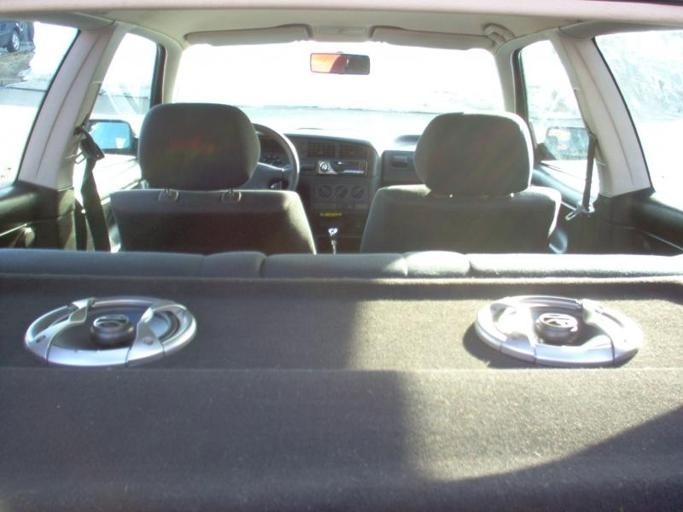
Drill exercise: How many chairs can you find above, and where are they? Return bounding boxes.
[359,111,563,255]
[108,100,313,257]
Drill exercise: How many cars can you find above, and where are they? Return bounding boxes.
[0,16,35,53]
